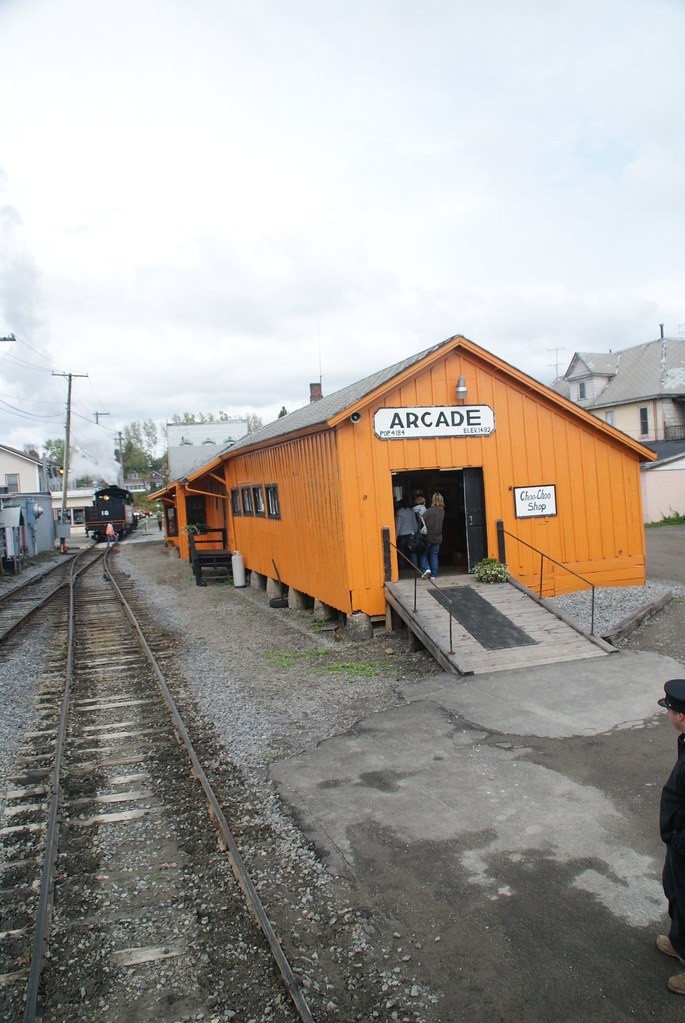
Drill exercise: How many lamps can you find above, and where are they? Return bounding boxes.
[455,377,467,400]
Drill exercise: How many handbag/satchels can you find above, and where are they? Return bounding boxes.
[408,519,428,554]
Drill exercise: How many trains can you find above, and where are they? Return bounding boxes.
[84,485,134,541]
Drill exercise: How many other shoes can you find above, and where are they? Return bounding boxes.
[667,974,685,994]
[431,574,435,579]
[656,934,685,965]
[421,568,431,579]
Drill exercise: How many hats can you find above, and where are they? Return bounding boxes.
[657,679,685,714]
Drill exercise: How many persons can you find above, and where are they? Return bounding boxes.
[394,499,417,571]
[420,493,445,580]
[656,679,685,995]
[412,497,427,515]
[106,521,113,547]
[157,511,162,531]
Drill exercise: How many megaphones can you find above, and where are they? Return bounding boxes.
[350,411,361,423]
